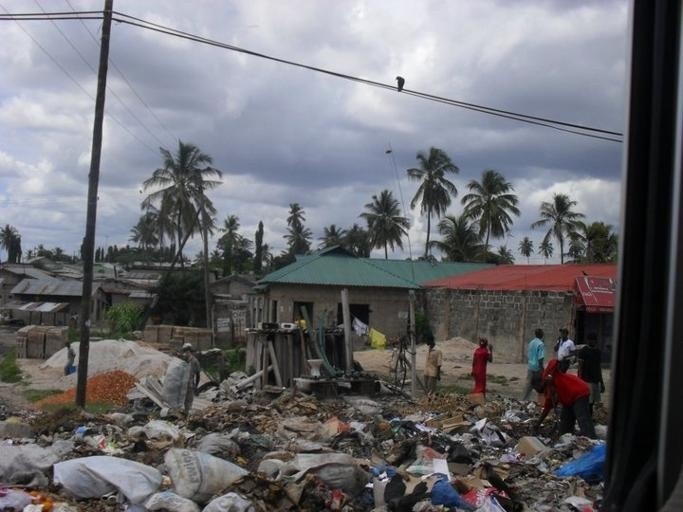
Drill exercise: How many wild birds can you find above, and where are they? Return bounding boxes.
[581,270,592,276]
[384,472,405,502]
[455,402,506,451]
[394,75,405,93]
[479,460,514,498]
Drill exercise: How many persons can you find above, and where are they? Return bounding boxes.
[518,326,545,407]
[528,368,597,443]
[551,327,573,373]
[422,338,443,394]
[537,357,570,407]
[468,335,493,399]
[60,341,76,376]
[181,341,202,420]
[574,333,605,419]
